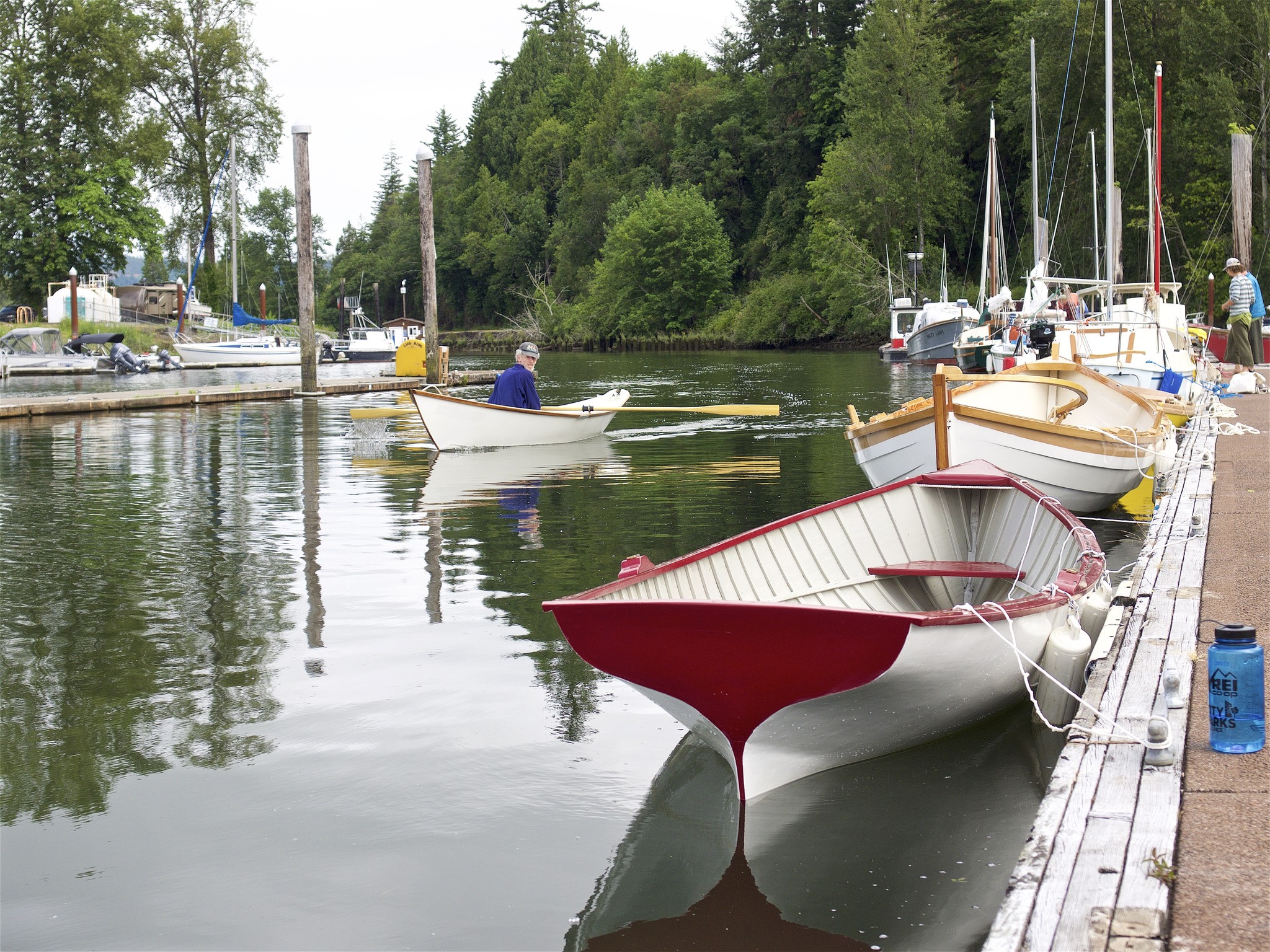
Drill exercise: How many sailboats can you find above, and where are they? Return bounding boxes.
[164,137,340,369]
[876,0,1221,419]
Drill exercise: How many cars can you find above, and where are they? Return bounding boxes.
[0,304,38,322]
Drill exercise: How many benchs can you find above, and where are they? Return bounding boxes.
[868,561,1026,580]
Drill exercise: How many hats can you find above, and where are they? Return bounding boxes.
[1060,284,1073,292]
[519,342,539,359]
[1222,257,1241,271]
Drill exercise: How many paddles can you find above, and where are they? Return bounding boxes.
[349,408,418,420]
[540,405,779,416]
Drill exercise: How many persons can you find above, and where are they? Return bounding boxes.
[1009,314,1027,346]
[1221,257,1267,375]
[487,342,541,411]
[1054,285,1089,322]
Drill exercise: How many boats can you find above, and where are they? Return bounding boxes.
[0,327,99,376]
[314,270,410,363]
[524,459,1113,804]
[133,345,185,372]
[845,340,1180,526]
[409,387,632,454]
[62,332,150,376]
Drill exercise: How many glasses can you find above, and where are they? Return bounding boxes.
[1225,268,1229,273]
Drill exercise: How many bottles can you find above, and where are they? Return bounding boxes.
[1194,618,1266,754]
